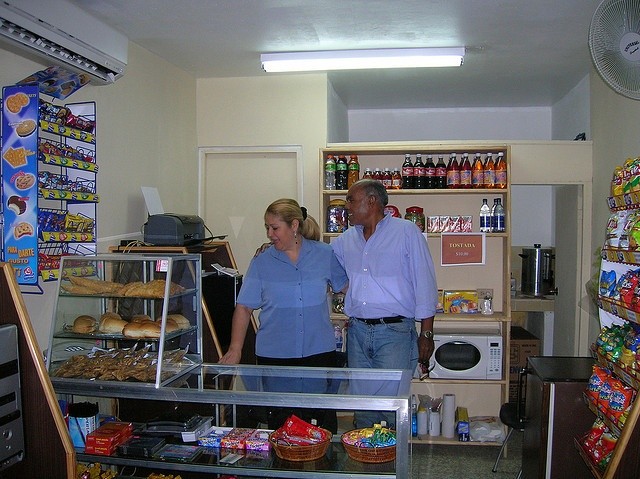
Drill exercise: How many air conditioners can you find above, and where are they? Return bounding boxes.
[1,1,129,86]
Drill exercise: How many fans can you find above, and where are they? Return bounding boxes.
[588,0,640,100]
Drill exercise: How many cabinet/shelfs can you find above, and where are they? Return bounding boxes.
[44,253,202,389]
[318,145,512,460]
[109,240,259,366]
[570,156,640,475]
[1,84,98,293]
[52,362,413,479]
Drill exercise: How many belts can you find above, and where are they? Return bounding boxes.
[356,315,408,324]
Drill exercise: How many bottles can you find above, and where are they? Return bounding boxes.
[424,155,436,188]
[402,154,414,188]
[447,152,459,188]
[324,155,336,189]
[335,154,348,189]
[460,152,472,188]
[363,167,401,189]
[484,152,496,188]
[435,154,447,189]
[479,198,491,233]
[496,152,507,188]
[414,154,425,188]
[347,155,359,189]
[473,152,484,188]
[491,198,505,232]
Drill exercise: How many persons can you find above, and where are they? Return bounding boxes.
[215,199,348,471]
[253,179,438,432]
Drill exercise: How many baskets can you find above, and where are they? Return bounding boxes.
[268,426,332,462]
[340,427,396,464]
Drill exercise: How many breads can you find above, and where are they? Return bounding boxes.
[6,195,26,217]
[70,309,191,339]
[61,273,186,298]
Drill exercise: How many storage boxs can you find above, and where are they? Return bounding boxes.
[517,356,639,478]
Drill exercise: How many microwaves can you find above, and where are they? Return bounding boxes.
[428,327,503,380]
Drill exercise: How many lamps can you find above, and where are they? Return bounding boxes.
[259,45,465,73]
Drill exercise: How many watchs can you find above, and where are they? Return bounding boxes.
[421,329,434,338]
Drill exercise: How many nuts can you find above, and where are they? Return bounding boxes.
[55,344,192,381]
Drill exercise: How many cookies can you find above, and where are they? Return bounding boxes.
[15,92,30,107]
[15,119,36,137]
[6,96,23,113]
[15,222,34,240]
[17,171,36,191]
[4,145,28,168]
